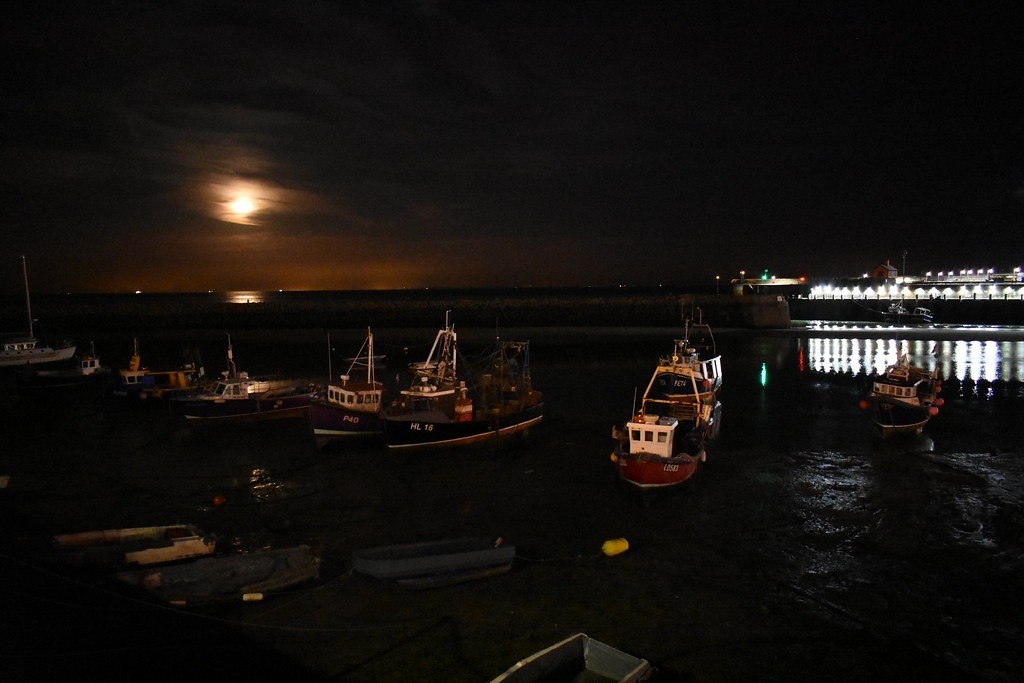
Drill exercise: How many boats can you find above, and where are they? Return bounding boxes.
[0,254,77,370]
[271,325,394,437]
[867,300,938,328]
[136,547,320,606]
[735,270,807,330]
[611,307,726,498]
[78,336,325,431]
[862,354,955,441]
[486,632,660,683]
[345,532,515,592]
[53,524,219,563]
[382,308,549,451]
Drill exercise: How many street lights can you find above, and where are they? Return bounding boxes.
[715,274,721,294]
